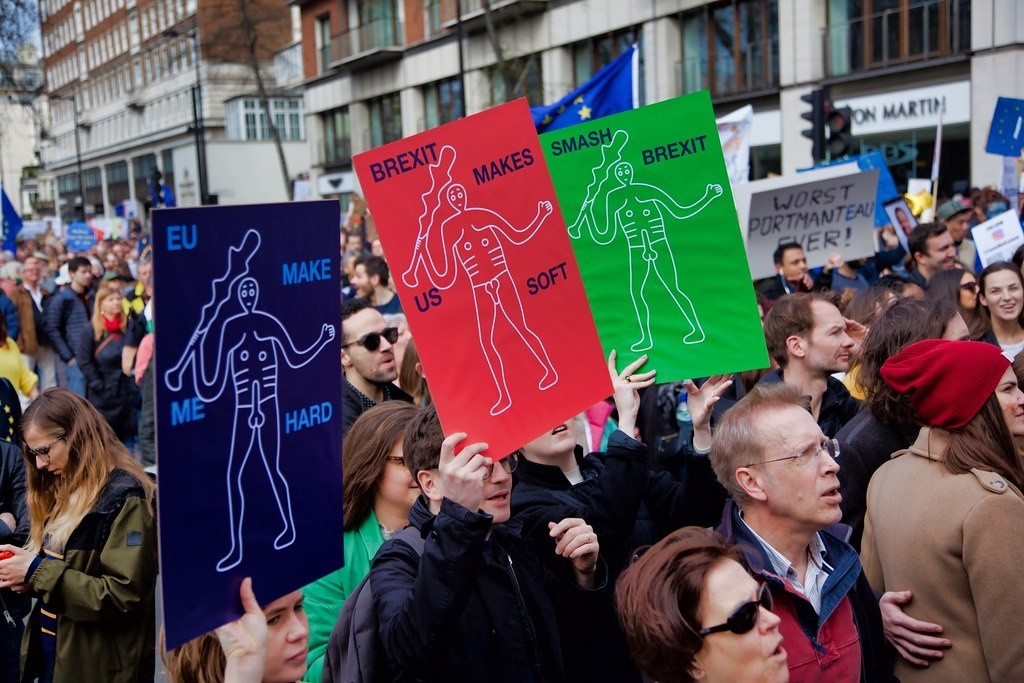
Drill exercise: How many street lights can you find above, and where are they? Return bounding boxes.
[161,28,209,207]
[48,92,85,223]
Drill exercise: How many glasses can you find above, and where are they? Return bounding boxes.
[698,582,773,636]
[733,438,840,475]
[29,432,66,455]
[340,327,399,352]
[416,449,519,486]
[959,282,977,293]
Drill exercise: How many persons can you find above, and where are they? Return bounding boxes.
[0,188,1024,683]
[894,207,913,236]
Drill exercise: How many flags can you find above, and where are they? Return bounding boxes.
[530,44,639,135]
[0,186,23,254]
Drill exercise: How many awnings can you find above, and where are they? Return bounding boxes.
[329,45,403,73]
[443,0,548,36]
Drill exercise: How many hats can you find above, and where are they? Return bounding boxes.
[880,338,1011,429]
[937,202,973,221]
[55,264,72,285]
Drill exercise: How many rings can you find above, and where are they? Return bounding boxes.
[586,534,590,543]
[626,376,631,382]
[712,392,714,397]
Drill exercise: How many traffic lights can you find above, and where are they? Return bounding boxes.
[801,88,831,163]
[828,106,853,163]
